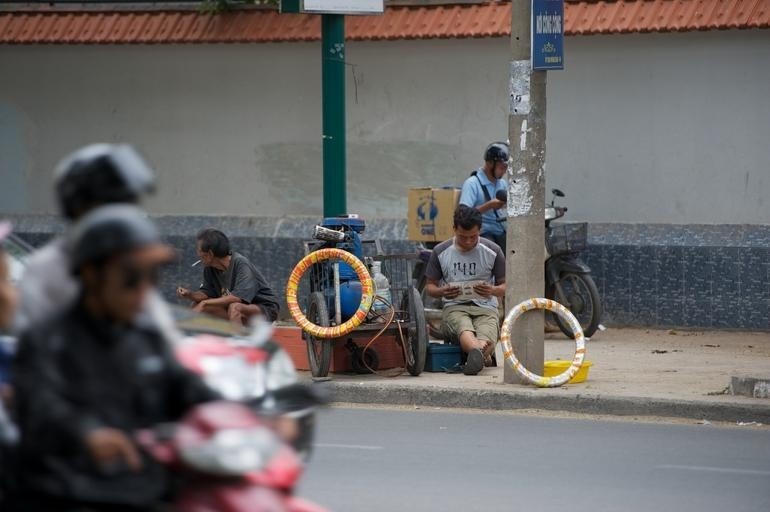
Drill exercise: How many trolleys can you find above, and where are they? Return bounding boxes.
[299,219,431,381]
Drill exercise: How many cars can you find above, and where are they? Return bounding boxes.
[2,232,327,467]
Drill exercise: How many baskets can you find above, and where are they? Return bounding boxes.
[544,222,588,257]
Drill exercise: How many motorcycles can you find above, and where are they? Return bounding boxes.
[409,188,603,338]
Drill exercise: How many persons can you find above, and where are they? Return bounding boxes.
[458,143,562,332]
[173,226,278,327]
[0,219,16,338]
[12,143,166,337]
[17,203,290,511]
[426,207,507,374]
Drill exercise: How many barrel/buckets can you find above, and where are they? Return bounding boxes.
[369,265,392,311]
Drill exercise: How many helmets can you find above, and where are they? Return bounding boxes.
[48,140,161,218]
[483,140,510,164]
[65,201,165,275]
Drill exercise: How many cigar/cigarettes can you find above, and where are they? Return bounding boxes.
[191,260,201,267]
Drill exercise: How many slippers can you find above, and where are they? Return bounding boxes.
[462,347,484,376]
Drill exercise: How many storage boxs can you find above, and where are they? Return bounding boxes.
[407,183,462,241]
